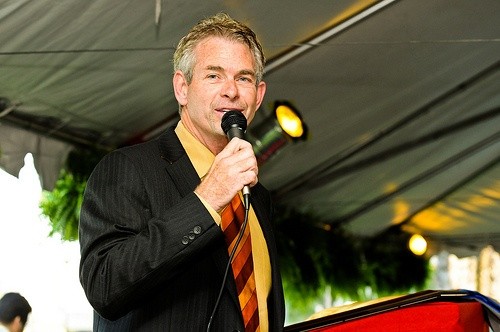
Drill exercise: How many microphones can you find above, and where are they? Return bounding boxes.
[220,111,249,197]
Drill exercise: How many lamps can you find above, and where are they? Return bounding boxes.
[242,99,308,167]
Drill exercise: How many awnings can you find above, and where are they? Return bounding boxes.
[0,0,500,265]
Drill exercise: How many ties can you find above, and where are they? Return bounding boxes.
[217,193,262,332]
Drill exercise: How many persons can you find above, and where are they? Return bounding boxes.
[0,293,33,332]
[78,12,287,331]
